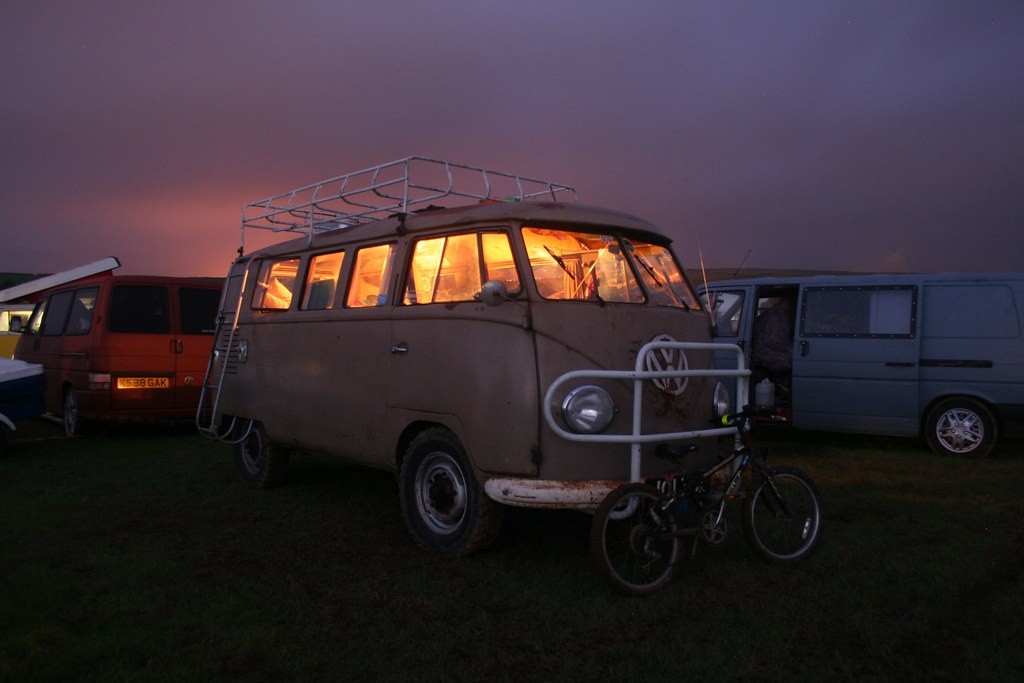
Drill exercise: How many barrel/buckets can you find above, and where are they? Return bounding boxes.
[755,379,775,406]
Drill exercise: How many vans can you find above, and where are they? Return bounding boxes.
[690,273,1024,461]
[195,158,729,554]
[10,272,230,436]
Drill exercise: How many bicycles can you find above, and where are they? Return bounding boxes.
[588,406,826,594]
